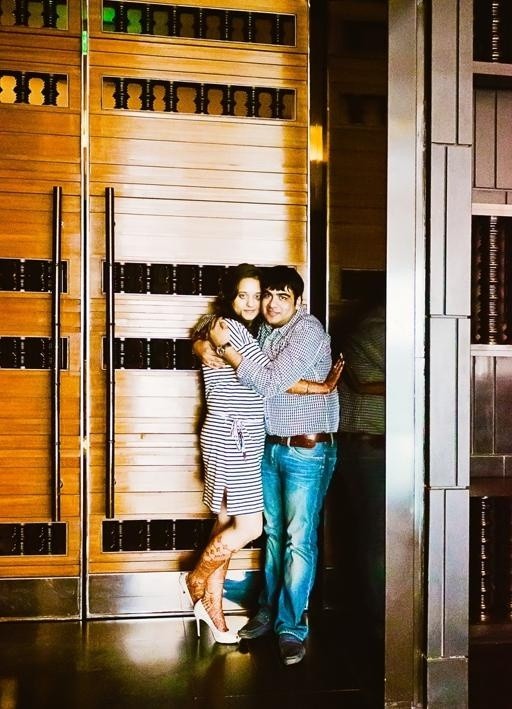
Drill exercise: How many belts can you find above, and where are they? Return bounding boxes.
[265,433,331,448]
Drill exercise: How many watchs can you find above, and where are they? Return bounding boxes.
[216,342,232,357]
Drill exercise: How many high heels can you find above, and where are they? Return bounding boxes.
[179,571,241,643]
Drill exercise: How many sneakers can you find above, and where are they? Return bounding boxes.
[238,608,275,640]
[278,634,306,666]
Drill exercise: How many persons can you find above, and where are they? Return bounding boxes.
[177,264,347,646]
[190,264,341,667]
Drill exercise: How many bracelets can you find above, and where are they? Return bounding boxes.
[307,381,310,394]
